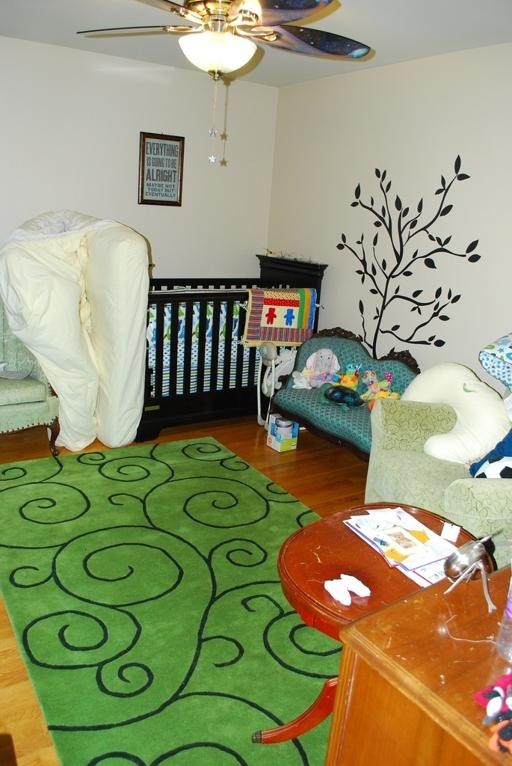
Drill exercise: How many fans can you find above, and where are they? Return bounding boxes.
[76,1,371,61]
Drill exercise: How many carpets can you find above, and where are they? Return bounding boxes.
[1,435,343,766]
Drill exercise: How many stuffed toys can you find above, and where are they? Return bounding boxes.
[358,370,393,403]
[261,347,298,398]
[368,388,402,412]
[300,348,341,389]
[330,364,361,392]
[291,370,313,390]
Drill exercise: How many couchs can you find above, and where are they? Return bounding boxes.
[272,326,422,463]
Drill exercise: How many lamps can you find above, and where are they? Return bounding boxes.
[256,342,282,432]
[177,31,259,164]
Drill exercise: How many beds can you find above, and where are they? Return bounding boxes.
[136,255,329,444]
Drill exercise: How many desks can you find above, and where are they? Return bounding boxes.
[250,502,494,743]
[324,563,512,766]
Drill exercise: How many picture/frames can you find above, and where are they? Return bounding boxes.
[137,131,184,207]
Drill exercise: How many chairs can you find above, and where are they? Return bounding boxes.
[364,397,512,570]
[0,302,60,457]
